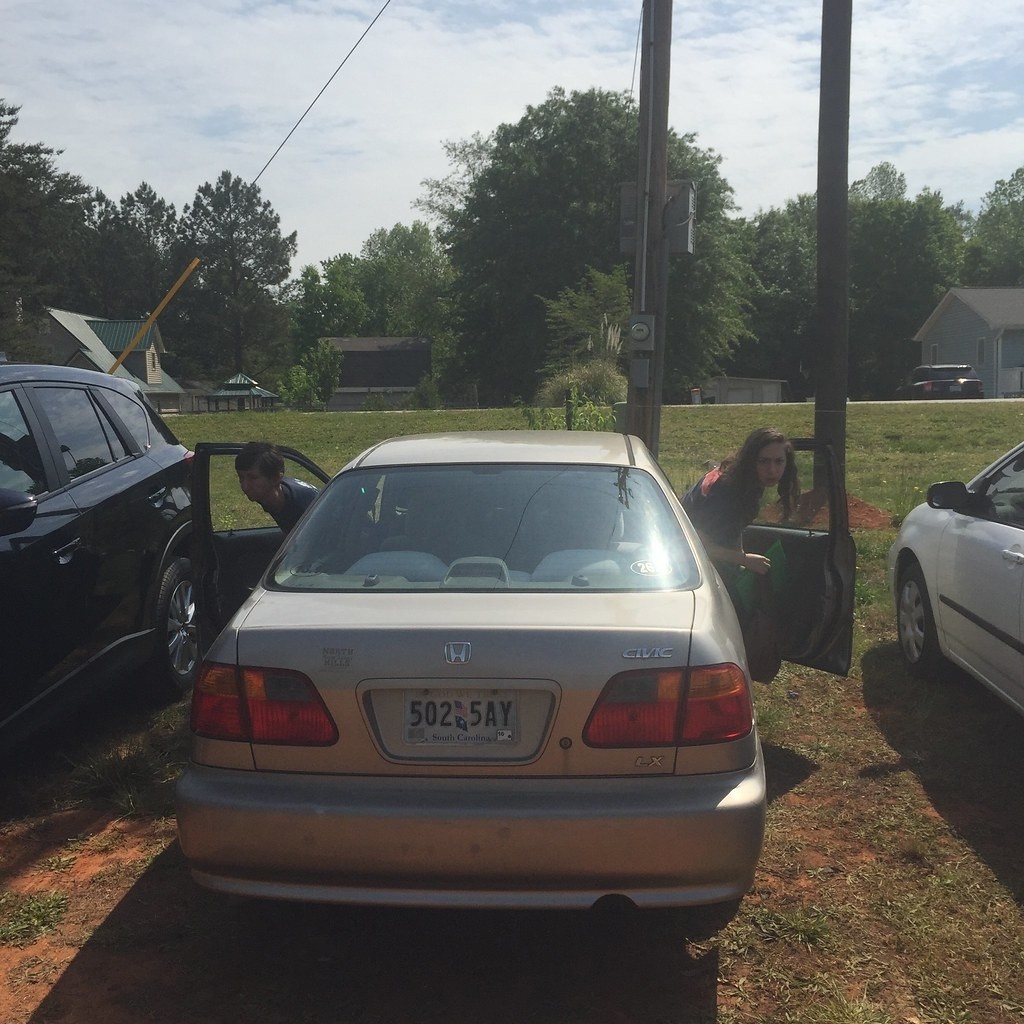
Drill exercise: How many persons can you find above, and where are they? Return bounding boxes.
[233,442,321,537]
[677,426,802,611]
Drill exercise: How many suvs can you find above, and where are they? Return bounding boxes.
[894,362,985,399]
[0,364,204,763]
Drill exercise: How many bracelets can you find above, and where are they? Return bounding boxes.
[741,553,747,566]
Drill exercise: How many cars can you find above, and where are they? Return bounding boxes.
[173,429,856,915]
[887,437,1024,716]
